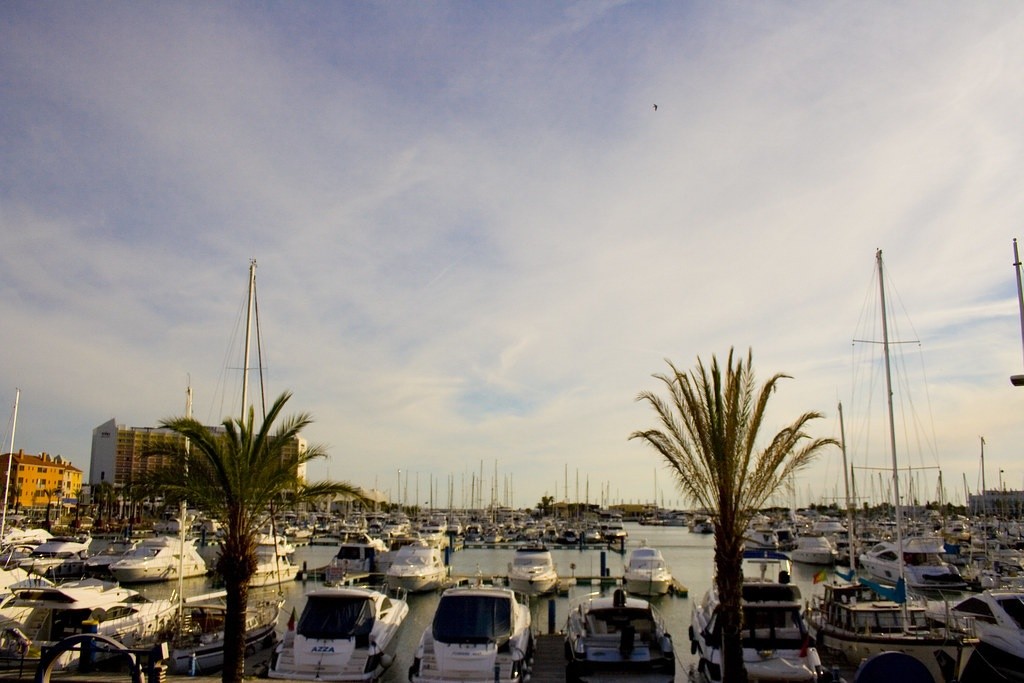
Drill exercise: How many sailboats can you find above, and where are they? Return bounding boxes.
[802,250,981,682]
[687,403,1024,592]
[152,387,285,676]
[283,459,628,552]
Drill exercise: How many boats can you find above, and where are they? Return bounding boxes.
[82,537,141,574]
[386,538,452,592]
[622,538,672,596]
[247,525,301,586]
[19,536,92,578]
[337,524,390,570]
[408,566,537,683]
[108,536,209,582]
[0,576,177,670]
[267,584,409,682]
[561,588,675,683]
[689,578,833,683]
[912,591,1024,671]
[506,538,558,595]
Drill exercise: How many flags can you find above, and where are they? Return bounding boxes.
[813,569,828,584]
